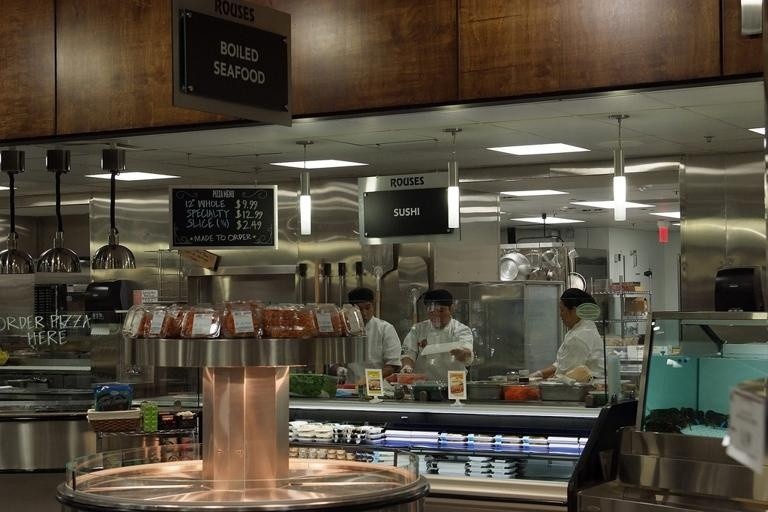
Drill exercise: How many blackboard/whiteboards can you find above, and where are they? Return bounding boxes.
[168,184,278,250]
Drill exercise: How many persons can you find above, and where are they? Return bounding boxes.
[327,287,402,379]
[525,288,605,381]
[400,289,475,379]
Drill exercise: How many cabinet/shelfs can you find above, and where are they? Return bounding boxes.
[586,275,654,360]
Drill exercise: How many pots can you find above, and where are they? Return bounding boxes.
[497,246,588,294]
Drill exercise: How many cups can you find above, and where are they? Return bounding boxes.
[519,369,530,383]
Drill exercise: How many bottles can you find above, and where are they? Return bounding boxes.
[506,368,517,380]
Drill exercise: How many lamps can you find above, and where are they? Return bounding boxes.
[606,114,637,221]
[295,139,314,236]
[444,127,463,228]
[0,148,142,273]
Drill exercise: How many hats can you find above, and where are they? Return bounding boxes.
[348,287,374,304]
[561,288,597,307]
[423,289,452,306]
